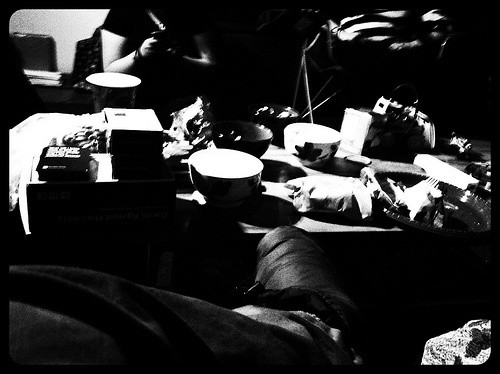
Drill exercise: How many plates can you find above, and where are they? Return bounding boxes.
[366,171,492,238]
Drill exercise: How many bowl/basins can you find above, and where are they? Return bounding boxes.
[247,103,299,142]
[283,122,342,170]
[212,121,273,160]
[187,147,265,212]
[241,161,308,215]
[86,72,143,112]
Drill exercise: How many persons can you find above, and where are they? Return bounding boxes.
[8,225,370,366]
[99,9,218,106]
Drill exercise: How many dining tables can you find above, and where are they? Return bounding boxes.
[9,113,493,240]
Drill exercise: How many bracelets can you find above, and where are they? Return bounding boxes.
[133,46,146,66]
